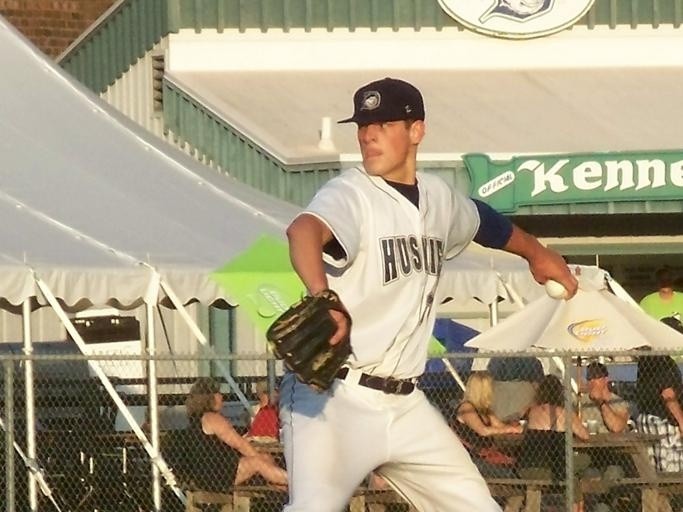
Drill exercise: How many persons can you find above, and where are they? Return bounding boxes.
[267,78,578,512]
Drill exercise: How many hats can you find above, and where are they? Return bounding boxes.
[586,362,608,379]
[337,77,425,123]
[650,264,679,282]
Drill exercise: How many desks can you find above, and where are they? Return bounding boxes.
[489,431,671,478]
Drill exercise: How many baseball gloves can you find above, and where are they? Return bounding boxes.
[266,289,357,393]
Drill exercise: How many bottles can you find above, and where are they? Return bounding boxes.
[587,414,598,436]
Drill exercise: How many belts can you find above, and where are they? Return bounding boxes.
[335,367,414,394]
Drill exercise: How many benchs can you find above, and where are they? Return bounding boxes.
[181,473,681,511]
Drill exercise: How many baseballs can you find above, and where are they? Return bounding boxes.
[545,279,568,299]
